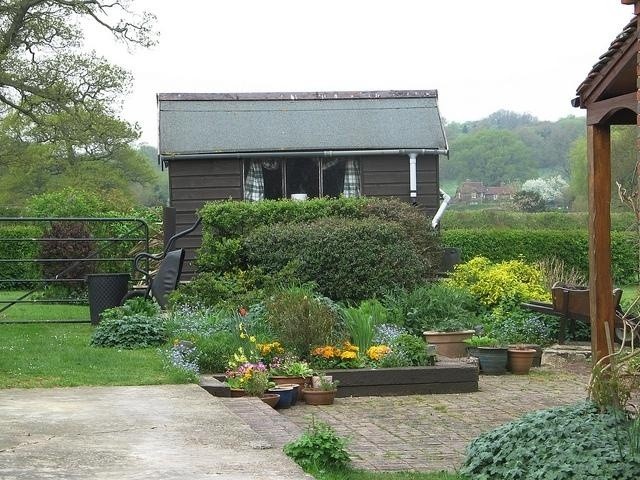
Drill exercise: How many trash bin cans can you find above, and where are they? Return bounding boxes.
[88,273,128,327]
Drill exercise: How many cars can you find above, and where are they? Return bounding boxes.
[543,199,569,213]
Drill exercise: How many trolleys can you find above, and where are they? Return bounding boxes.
[521,281,640,350]
[119,215,202,311]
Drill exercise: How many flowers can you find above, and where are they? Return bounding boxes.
[270,352,314,376]
[225,349,268,394]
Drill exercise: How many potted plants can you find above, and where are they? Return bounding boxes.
[420,295,543,375]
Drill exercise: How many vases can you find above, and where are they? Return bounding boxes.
[230,376,340,410]
